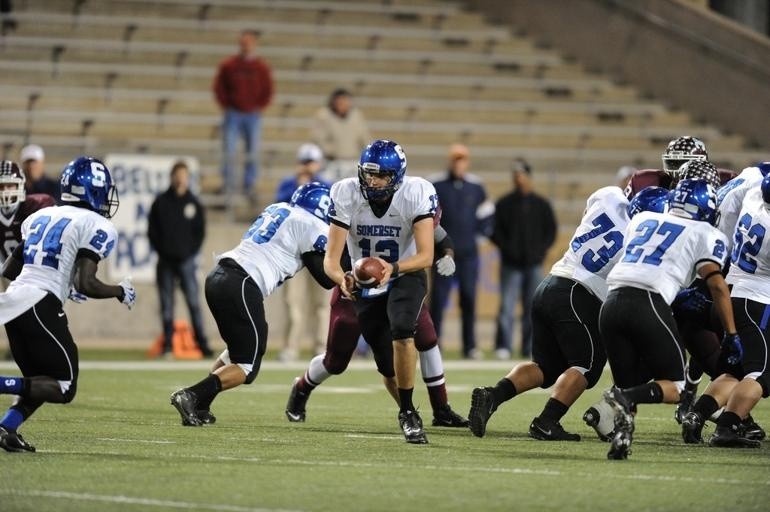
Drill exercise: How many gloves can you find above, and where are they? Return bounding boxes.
[435,255,456,277]
[68,285,89,304]
[720,331,745,365]
[675,286,709,315]
[117,275,136,310]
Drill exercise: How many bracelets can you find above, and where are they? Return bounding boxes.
[390,263,399,278]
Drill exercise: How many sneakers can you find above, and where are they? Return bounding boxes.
[675,386,765,448]
[397,387,493,444]
[0,424,35,452]
[286,376,309,421]
[529,417,581,442]
[169,387,203,426]
[196,407,216,423]
[583,383,635,459]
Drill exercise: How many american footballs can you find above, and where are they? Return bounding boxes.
[352,257,385,288]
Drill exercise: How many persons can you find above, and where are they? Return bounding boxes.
[413,211,470,427]
[467,134,770,463]
[209,26,275,207]
[0,158,57,284]
[15,144,66,207]
[169,181,350,425]
[308,86,370,182]
[430,147,493,359]
[276,143,334,359]
[0,155,135,455]
[146,158,219,364]
[287,138,439,444]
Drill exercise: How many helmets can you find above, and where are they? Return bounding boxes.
[60,155,119,218]
[290,181,337,225]
[357,140,407,203]
[0,160,28,217]
[627,136,770,220]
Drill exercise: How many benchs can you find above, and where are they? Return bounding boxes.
[0,0,770,232]
